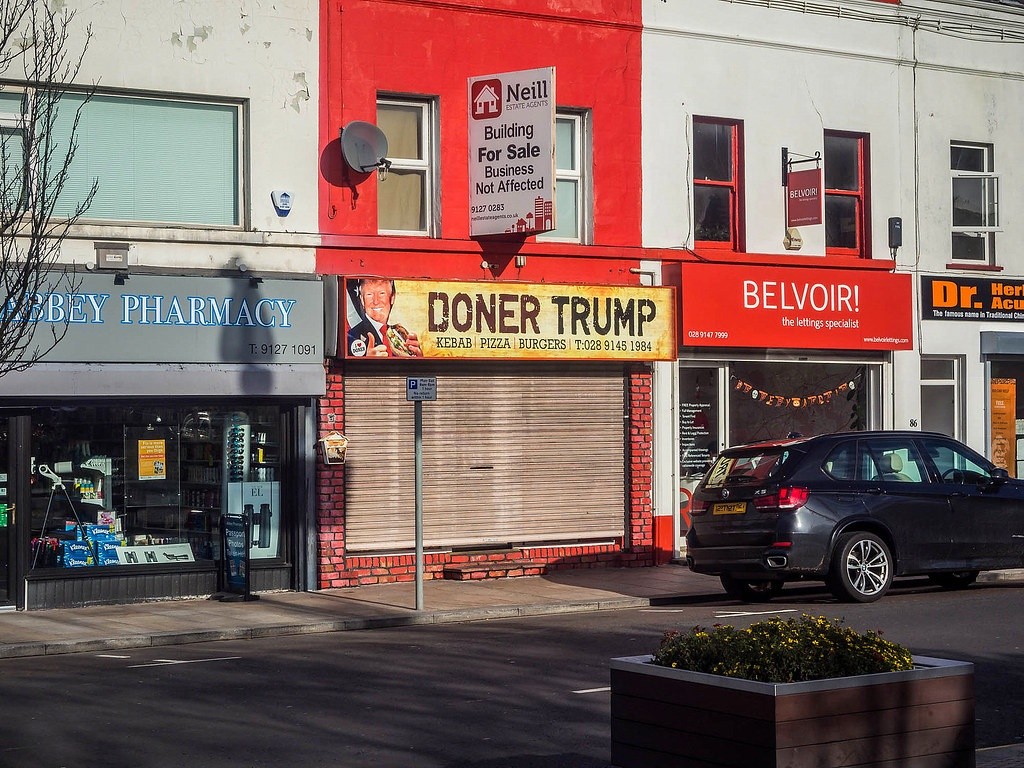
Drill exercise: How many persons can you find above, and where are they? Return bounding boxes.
[348,279,422,358]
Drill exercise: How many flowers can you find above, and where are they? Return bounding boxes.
[650,613,915,683]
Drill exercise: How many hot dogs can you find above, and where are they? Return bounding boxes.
[384,325,415,357]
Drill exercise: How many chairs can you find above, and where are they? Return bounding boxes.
[873,453,916,482]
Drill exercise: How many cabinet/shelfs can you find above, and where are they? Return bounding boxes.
[0,408,286,568]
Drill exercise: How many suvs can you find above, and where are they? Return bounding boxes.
[685,430,1024,602]
